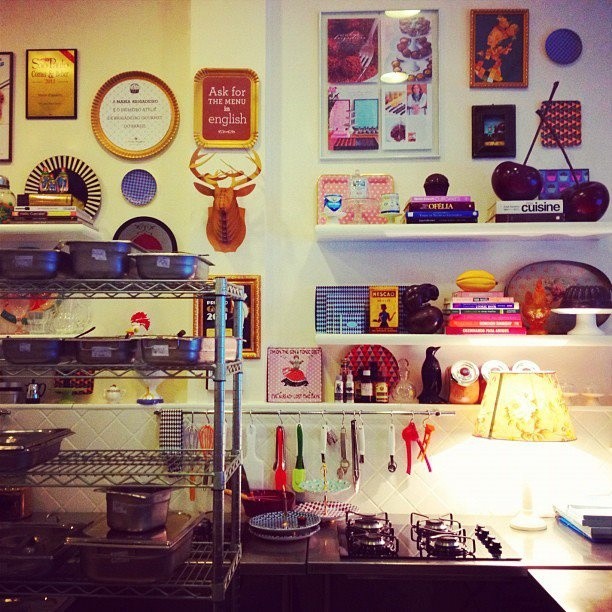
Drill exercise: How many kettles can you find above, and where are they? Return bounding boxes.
[24,379,46,404]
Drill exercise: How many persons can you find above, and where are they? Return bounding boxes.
[373,304,396,327]
[280,354,309,389]
[407,84,427,115]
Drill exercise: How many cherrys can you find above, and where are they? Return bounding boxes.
[492,81,559,200]
[534,109,609,221]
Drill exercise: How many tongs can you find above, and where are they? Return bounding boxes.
[272,424,287,490]
[351,420,360,494]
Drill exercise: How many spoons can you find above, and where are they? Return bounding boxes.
[388,423,397,473]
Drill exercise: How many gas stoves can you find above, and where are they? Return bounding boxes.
[336,510,522,561]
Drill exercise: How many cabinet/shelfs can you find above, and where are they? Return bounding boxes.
[315,221,611,349]
[0,272,246,612]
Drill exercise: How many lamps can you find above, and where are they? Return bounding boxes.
[472,370,578,533]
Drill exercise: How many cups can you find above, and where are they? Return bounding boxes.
[51,294,91,334]
[27,311,47,334]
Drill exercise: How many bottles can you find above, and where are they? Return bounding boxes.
[353,369,363,403]
[346,370,355,403]
[390,359,416,403]
[375,371,389,403]
[334,368,343,403]
[361,370,373,403]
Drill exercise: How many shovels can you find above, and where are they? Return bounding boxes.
[275,428,286,491]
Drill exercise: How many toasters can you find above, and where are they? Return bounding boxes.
[0,482,33,522]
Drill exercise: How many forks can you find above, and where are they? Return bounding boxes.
[355,18,378,82]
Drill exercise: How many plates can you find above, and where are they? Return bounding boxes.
[113,216,177,253]
[24,155,101,219]
[342,345,400,397]
[121,169,157,206]
[248,525,320,541]
[299,479,351,496]
[248,511,321,532]
[293,500,359,521]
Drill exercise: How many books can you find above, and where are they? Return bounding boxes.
[400,196,478,223]
[486,199,566,222]
[554,501,612,542]
[12,192,100,231]
[441,291,527,333]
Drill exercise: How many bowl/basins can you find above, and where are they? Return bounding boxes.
[241,489,294,518]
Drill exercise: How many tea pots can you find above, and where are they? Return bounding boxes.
[102,384,126,404]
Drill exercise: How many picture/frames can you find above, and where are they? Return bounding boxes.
[25,49,77,119]
[318,9,444,160]
[91,71,180,160]
[0,52,13,162]
[193,68,259,150]
[470,8,529,89]
[471,104,516,159]
[192,275,261,359]
